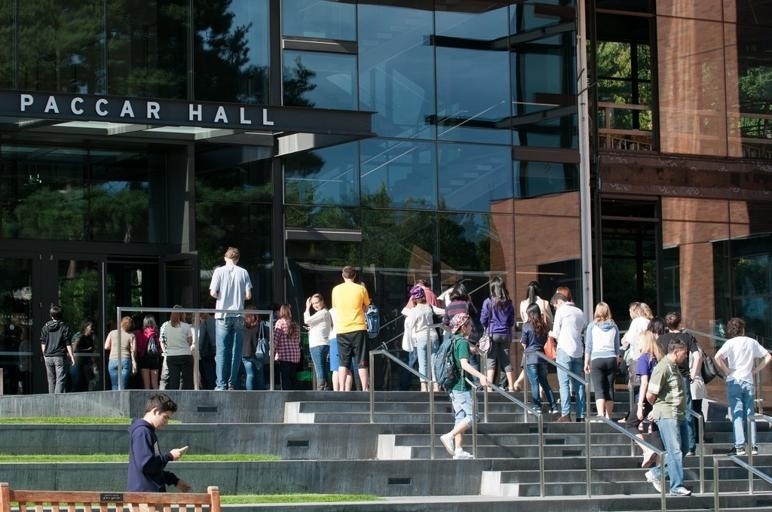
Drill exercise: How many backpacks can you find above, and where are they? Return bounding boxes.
[364,304,381,339]
[144,334,161,358]
[432,337,463,391]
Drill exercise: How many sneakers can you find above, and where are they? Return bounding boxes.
[685,449,696,457]
[528,405,647,426]
[215,382,237,392]
[726,445,758,456]
[453,451,476,461]
[644,470,667,494]
[439,432,457,456]
[315,382,331,390]
[639,451,657,468]
[476,380,523,392]
[669,486,693,497]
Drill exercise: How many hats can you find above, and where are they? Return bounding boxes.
[449,312,470,334]
[409,286,426,300]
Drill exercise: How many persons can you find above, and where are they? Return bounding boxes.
[39,305,76,394]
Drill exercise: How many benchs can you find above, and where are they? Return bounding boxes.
[1,480,220,511]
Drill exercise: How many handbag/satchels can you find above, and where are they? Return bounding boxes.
[541,335,559,359]
[255,321,272,359]
[700,345,719,385]
[477,330,494,356]
